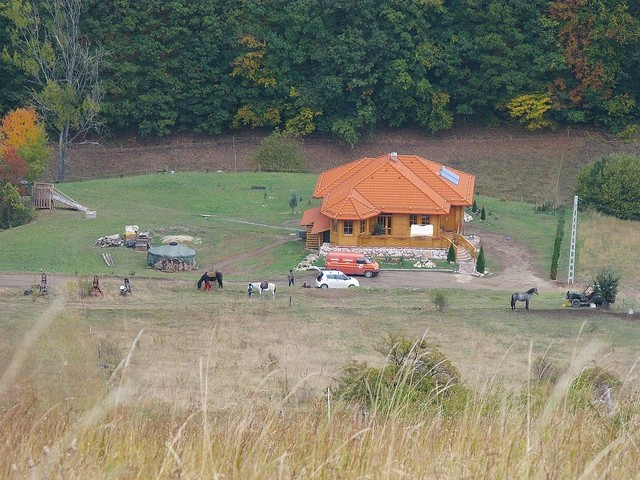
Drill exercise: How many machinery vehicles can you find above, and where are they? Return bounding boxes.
[566,284,616,308]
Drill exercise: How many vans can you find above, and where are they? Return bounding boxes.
[325,252,380,278]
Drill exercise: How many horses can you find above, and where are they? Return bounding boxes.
[511,288,539,311]
[247,281,277,300]
[197,272,223,290]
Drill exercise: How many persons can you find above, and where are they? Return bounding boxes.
[204,271,210,290]
[288,270,294,287]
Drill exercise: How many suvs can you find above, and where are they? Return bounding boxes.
[314,270,360,289]
